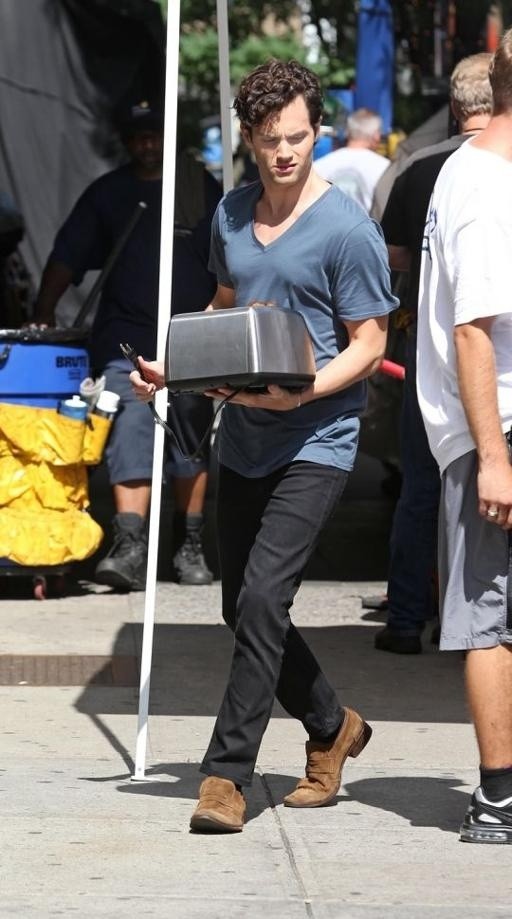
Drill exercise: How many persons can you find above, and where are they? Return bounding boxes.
[411,25,512,846]
[23,97,226,595]
[351,51,498,612]
[315,105,393,217]
[371,145,499,658]
[124,60,403,835]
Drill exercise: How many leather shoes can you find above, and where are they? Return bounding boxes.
[280,700,372,811]
[186,774,247,835]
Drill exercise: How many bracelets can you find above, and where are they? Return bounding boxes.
[295,391,302,408]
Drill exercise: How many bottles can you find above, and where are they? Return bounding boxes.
[61,390,121,424]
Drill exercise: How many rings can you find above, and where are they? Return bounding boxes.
[488,510,498,518]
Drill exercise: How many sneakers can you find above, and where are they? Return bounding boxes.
[166,515,213,586]
[94,510,148,590]
[456,786,512,845]
[355,587,423,657]
